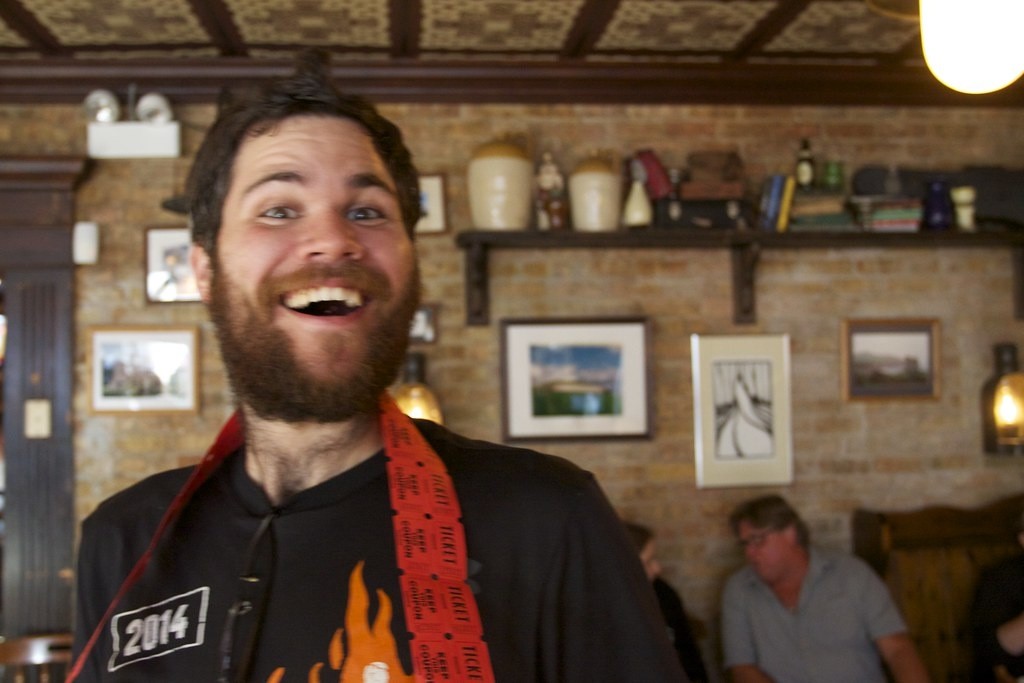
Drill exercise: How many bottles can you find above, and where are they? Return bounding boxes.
[535,152,567,233]
[925,179,954,230]
[797,139,815,194]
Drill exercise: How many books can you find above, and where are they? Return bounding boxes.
[753,176,927,235]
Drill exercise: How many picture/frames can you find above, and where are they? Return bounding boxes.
[690,329,801,490]
[83,322,205,418]
[409,174,451,235]
[835,312,943,404]
[500,313,652,448]
[142,225,205,307]
[408,300,441,344]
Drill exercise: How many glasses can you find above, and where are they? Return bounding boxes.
[736,525,780,549]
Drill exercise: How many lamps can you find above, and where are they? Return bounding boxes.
[982,340,1024,452]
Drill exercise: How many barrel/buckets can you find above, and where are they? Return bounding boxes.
[468,143,532,232]
[568,157,622,234]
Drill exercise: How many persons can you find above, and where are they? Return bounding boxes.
[975,510,1024,683]
[718,492,929,683]
[619,520,706,682]
[66,75,689,683]
